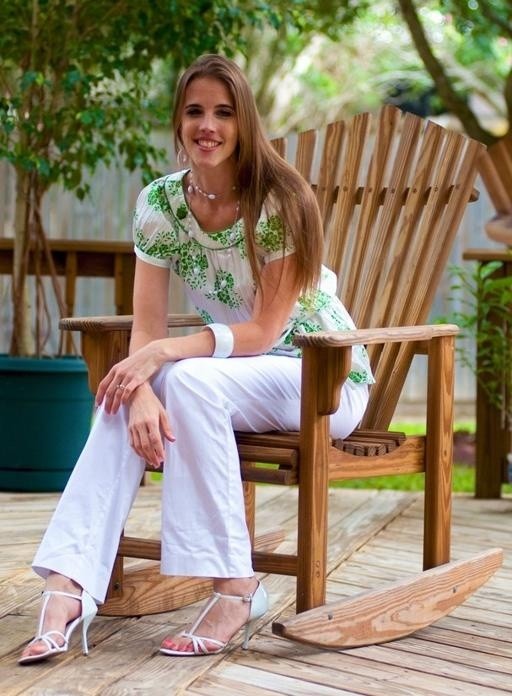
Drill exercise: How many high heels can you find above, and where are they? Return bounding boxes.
[18,589,98,662]
[158,580,268,656]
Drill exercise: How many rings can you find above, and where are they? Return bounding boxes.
[116,384,128,390]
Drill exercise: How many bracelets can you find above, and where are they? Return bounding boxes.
[199,320,237,362]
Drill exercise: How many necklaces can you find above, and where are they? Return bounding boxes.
[185,169,240,202]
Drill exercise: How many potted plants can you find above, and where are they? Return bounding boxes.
[0,0,257,494]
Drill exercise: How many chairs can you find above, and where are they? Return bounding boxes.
[60,102,503,650]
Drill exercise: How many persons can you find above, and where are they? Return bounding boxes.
[13,52,377,665]
